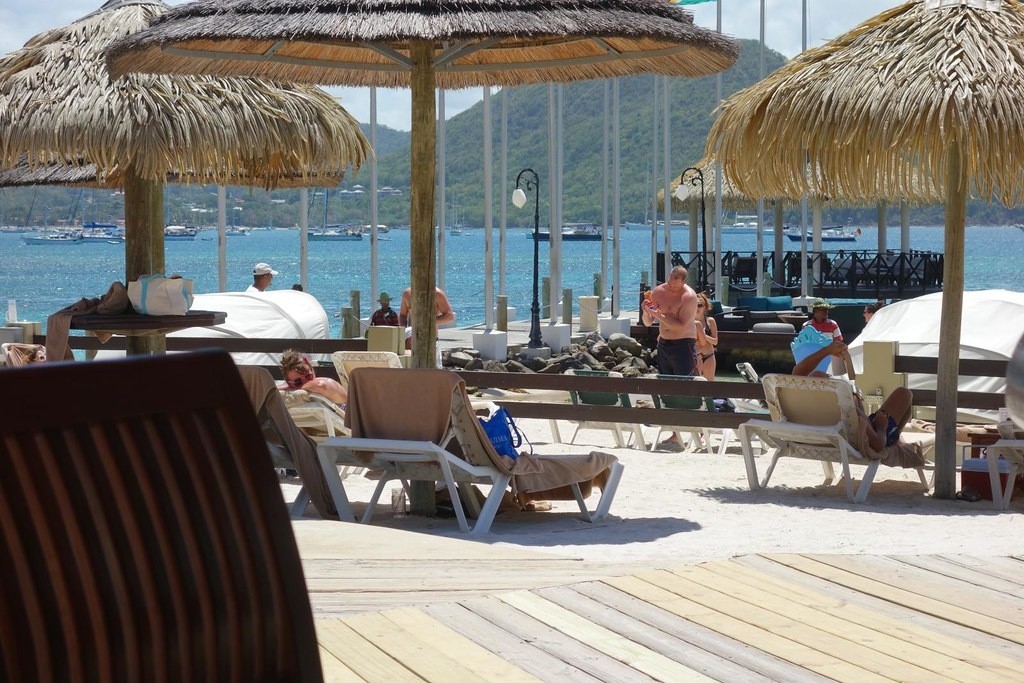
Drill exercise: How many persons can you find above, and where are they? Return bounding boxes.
[692,294,718,378]
[641,266,706,449]
[792,341,913,453]
[802,300,844,376]
[863,304,879,322]
[292,284,304,292]
[877,297,888,310]
[369,292,399,327]
[277,349,349,413]
[398,286,454,370]
[246,263,278,293]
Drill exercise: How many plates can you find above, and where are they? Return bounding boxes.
[984,427,999,433]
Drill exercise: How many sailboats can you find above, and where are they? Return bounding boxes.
[308,187,363,241]
[18,185,125,245]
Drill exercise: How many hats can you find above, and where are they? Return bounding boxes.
[809,297,836,309]
[252,263,278,275]
[377,292,393,302]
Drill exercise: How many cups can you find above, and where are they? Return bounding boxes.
[391,488,406,519]
[999,408,1008,423]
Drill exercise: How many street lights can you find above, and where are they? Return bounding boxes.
[675,166,712,300]
[510,166,545,348]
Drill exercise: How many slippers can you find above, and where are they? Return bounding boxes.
[662,437,677,444]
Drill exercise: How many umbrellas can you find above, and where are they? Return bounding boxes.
[105,0,740,369]
[657,144,945,290]
[704,0,1024,500]
[0,1,376,355]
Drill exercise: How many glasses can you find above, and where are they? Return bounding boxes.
[863,309,870,314]
[697,304,706,308]
[289,373,311,388]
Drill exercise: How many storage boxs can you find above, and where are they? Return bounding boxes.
[368,325,406,354]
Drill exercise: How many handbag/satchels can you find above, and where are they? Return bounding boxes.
[714,397,737,412]
[127,274,194,315]
[478,406,522,460]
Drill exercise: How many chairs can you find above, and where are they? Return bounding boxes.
[738,373,937,504]
[707,302,752,331]
[0,350,324,683]
[236,350,624,534]
[961,439,1024,509]
[565,363,768,456]
[732,251,936,287]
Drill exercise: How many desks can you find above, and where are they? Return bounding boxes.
[967,428,1024,458]
[71,310,227,357]
[777,311,808,332]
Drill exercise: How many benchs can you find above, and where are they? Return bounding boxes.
[753,323,795,332]
[731,296,807,328]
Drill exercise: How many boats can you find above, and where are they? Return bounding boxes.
[531,221,603,242]
[450,225,462,236]
[164,225,198,241]
[786,224,857,243]
[226,225,251,236]
